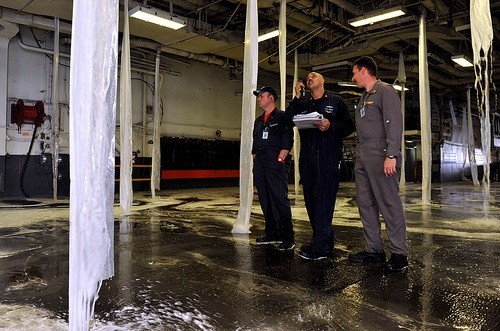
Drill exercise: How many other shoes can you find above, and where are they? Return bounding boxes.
[381,253,410,270]
[278,237,296,250]
[255,234,282,244]
[348,250,387,264]
[297,243,335,260]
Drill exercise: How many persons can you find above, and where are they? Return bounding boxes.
[285,71,354,261]
[349,56,409,271]
[251,86,295,250]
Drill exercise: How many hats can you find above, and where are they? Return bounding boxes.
[253,86,279,102]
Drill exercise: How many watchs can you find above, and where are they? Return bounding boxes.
[278,157,284,163]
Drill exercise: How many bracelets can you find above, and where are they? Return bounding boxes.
[387,156,397,159]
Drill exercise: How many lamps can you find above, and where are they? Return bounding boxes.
[348,5,407,27]
[451,55,474,67]
[243,26,280,44]
[128,0,188,30]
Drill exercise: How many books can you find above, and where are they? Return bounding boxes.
[293,111,323,129]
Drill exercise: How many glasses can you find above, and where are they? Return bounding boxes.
[359,101,364,111]
